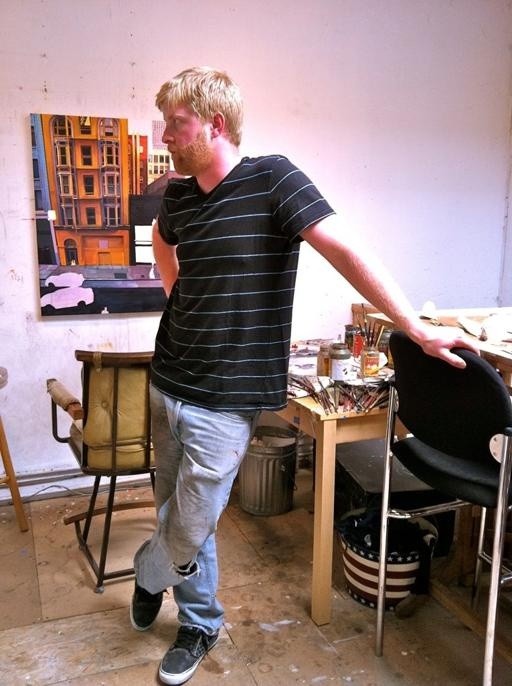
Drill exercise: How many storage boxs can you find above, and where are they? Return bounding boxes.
[314,439,455,559]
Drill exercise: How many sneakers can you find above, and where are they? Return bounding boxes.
[157,624,220,685]
[130,575,165,631]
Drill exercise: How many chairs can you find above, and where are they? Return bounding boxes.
[46,349,156,595]
[376,330,512,686]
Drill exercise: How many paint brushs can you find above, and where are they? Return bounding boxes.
[368,322,371,347]
[287,374,389,416]
[364,318,367,336]
[375,325,384,347]
[370,322,375,346]
[358,320,363,336]
[374,324,382,347]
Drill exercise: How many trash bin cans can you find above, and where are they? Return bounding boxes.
[237,426,299,517]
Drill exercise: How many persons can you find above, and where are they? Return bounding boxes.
[129,67,480,686]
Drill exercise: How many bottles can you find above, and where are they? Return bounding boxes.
[344,324,354,347]
[361,347,379,377]
[328,342,351,381]
[353,335,362,355]
[316,344,329,376]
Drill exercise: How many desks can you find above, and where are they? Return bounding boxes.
[273,338,472,627]
